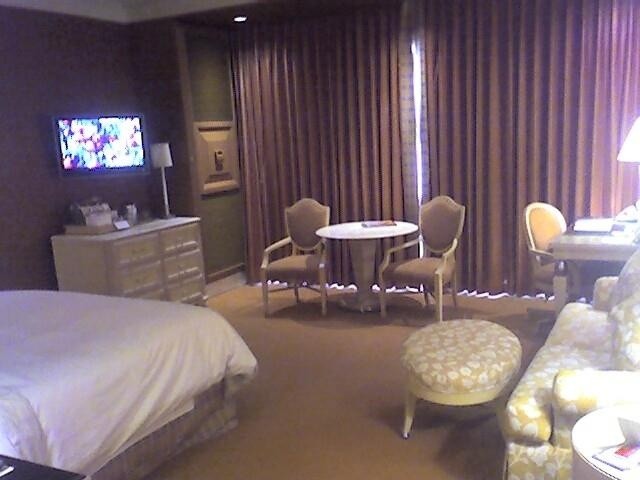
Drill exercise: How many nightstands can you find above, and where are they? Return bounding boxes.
[2,454,91,479]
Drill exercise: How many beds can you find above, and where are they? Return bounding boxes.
[1,287,257,479]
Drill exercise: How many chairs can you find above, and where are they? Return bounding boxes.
[260,198,330,317]
[523,201,568,327]
[378,194,467,322]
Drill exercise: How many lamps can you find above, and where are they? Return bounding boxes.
[147,142,174,220]
[616,116,640,162]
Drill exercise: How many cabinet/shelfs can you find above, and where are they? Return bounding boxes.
[50,216,211,306]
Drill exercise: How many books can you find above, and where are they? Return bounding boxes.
[574,218,615,233]
[362,220,398,227]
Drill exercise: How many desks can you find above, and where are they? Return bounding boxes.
[551,217,640,313]
[569,408,640,480]
[314,219,419,315]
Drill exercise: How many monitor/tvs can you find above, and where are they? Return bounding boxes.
[51,112,151,177]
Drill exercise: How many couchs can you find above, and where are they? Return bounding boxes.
[503,250,640,479]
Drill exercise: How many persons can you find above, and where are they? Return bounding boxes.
[57,117,144,168]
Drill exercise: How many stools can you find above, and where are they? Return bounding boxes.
[400,313,521,442]
